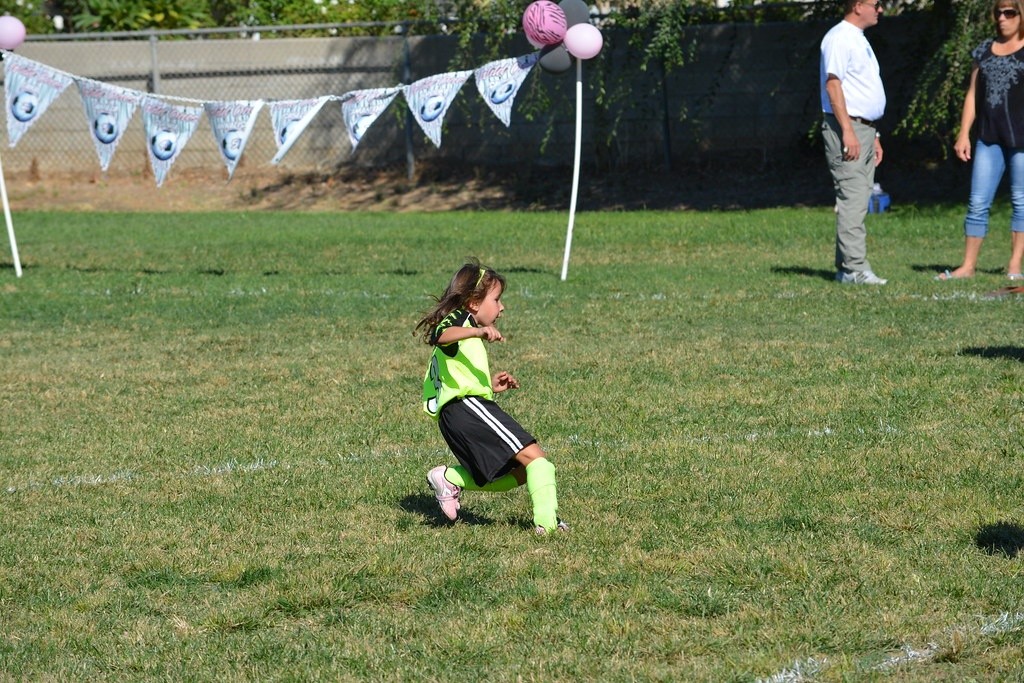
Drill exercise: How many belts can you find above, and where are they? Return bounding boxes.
[849,115,876,127]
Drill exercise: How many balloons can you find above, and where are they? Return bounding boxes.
[522,0,603,74]
[0,15,27,50]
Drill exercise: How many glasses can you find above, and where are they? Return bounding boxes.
[994,9,1021,20]
[860,2,883,13]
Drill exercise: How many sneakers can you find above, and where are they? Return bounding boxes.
[534,518,568,535]
[426,465,462,521]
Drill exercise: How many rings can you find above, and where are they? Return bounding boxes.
[843,147,849,153]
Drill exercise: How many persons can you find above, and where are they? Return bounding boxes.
[820,0,888,285]
[936,0,1024,280]
[414,256,570,539]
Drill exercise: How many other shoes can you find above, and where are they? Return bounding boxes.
[839,266,888,285]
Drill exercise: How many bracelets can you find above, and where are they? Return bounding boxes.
[875,132,881,138]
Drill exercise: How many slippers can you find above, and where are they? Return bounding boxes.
[1007,273,1024,282]
[935,270,950,280]
